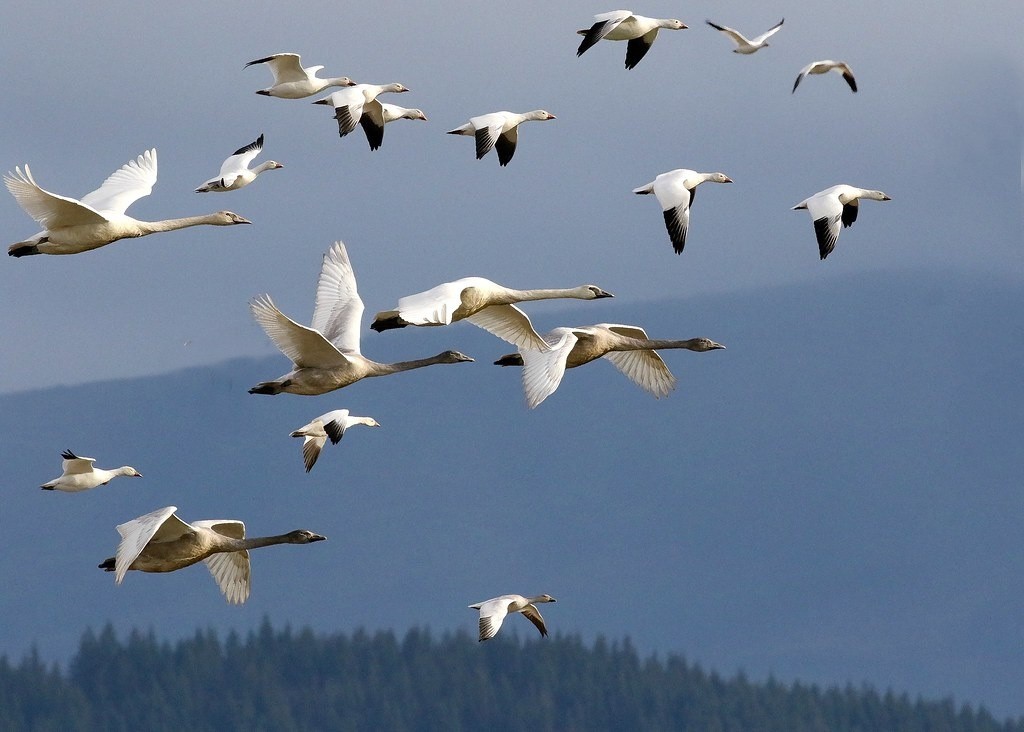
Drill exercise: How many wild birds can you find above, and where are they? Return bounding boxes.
[467,594,558,643]
[704,17,785,55]
[1,148,253,259]
[248,240,728,411]
[98,506,328,607]
[288,409,381,478]
[790,184,892,261]
[631,168,733,256]
[193,133,284,194]
[575,10,690,71]
[39,449,143,493]
[446,110,558,168]
[242,52,428,153]
[792,59,858,95]
